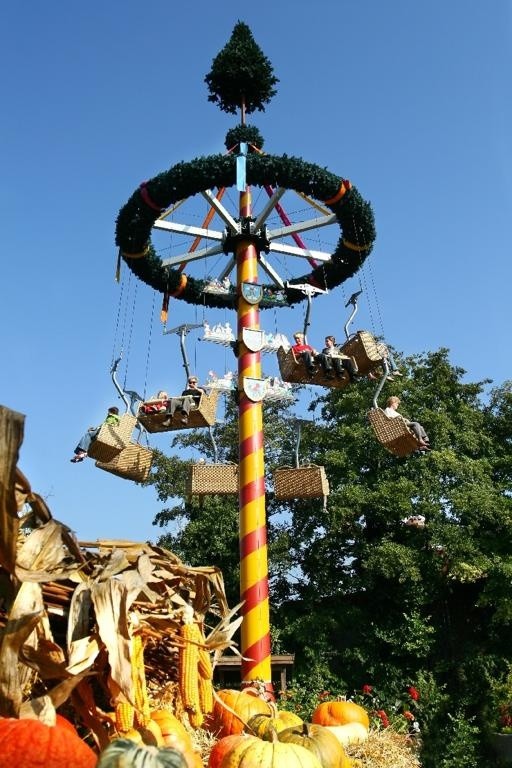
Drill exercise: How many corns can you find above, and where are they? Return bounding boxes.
[115,636,150,733]
[180,624,212,729]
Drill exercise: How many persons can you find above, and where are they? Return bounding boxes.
[382,352,404,383]
[69,405,120,463]
[322,336,362,381]
[367,368,382,381]
[384,395,432,453]
[290,332,332,377]
[140,390,168,415]
[161,375,207,429]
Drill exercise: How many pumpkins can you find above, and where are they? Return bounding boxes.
[0,689,369,768]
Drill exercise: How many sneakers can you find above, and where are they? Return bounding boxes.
[306,363,364,384]
[70,452,88,462]
[369,371,403,382]
[416,441,432,452]
[161,420,174,428]
[180,416,189,425]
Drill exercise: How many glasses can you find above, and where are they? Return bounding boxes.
[189,381,196,384]
